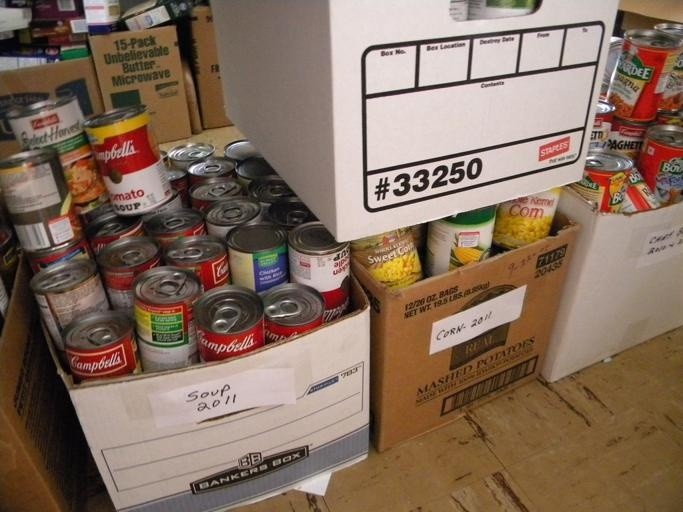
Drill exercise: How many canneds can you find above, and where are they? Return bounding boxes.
[451,1,536,22]
[493,187,563,251]
[569,22,683,215]
[0,94,351,380]
[351,227,425,292]
[426,206,496,278]
